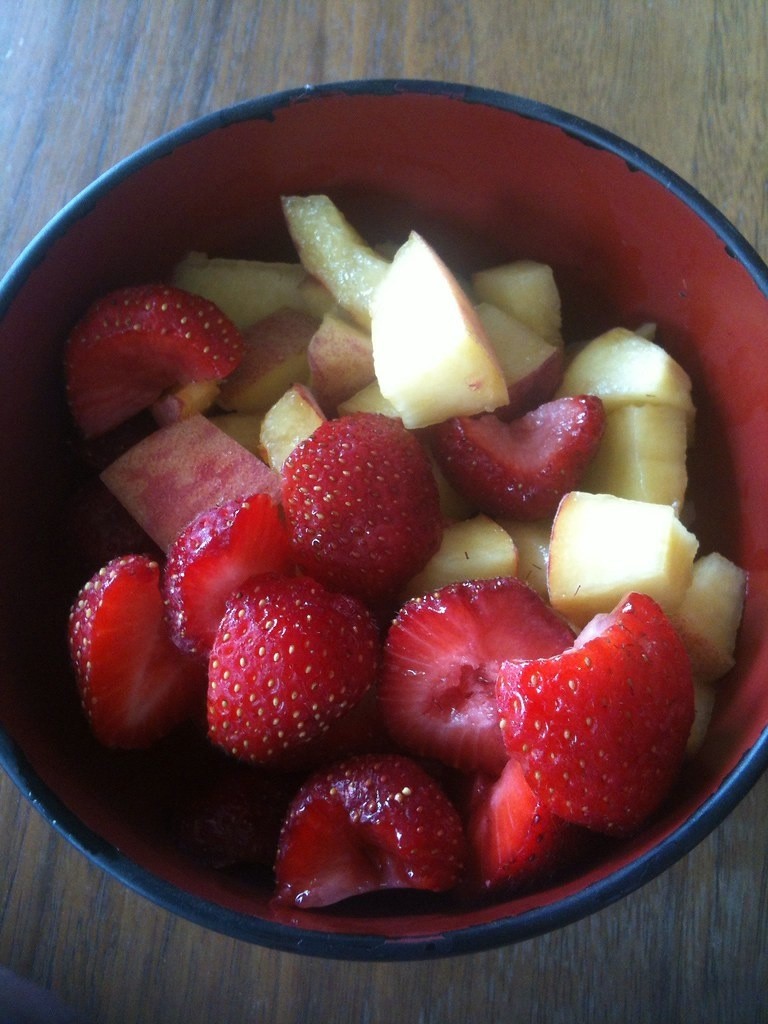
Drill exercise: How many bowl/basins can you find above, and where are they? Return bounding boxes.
[0,78,768,961]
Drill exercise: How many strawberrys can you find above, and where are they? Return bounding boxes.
[66,287,698,910]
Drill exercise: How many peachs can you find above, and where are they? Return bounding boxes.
[100,198,744,752]
